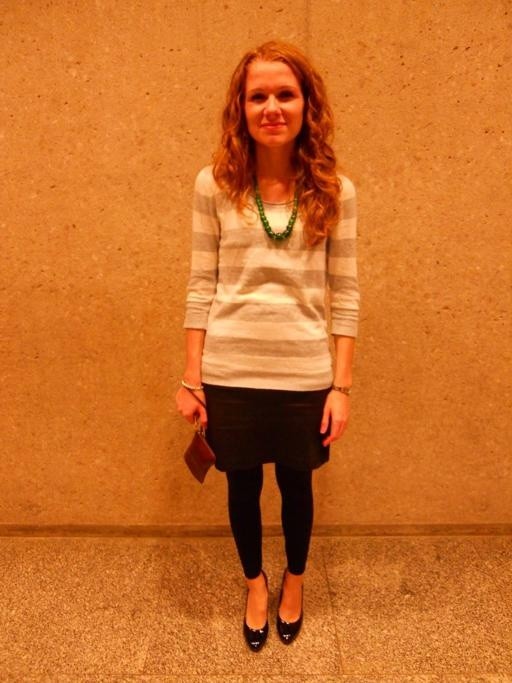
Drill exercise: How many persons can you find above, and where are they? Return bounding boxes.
[173,37,358,651]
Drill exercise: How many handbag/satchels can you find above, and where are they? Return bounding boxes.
[182,386,216,487]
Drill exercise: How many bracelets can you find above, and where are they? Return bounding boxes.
[330,382,353,396]
[181,379,205,391]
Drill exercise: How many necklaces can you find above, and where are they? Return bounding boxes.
[252,168,299,241]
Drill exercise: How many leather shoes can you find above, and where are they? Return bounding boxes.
[275,567,306,644]
[242,569,270,650]
